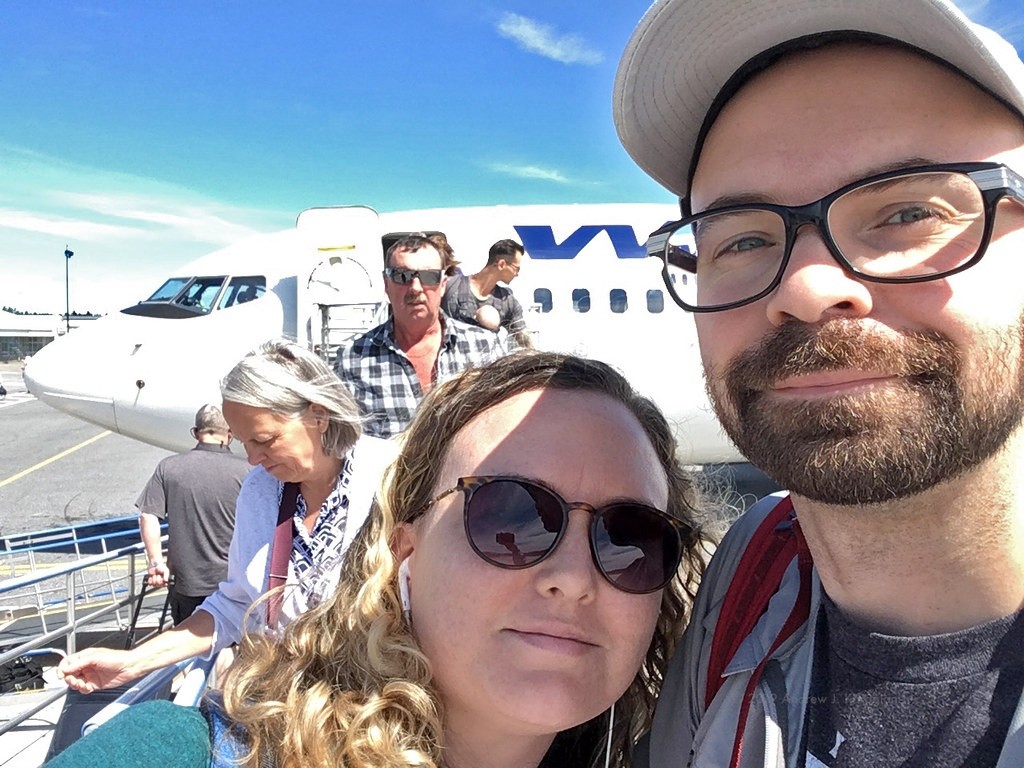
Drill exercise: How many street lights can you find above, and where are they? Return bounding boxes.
[65,249,74,332]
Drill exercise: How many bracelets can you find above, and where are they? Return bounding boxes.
[147,558,163,569]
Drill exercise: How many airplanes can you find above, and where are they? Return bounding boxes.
[19,202,749,466]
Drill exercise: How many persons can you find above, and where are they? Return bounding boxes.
[46,347,722,768]
[613,0,1024,768]
[333,233,537,439]
[238,285,258,304]
[21,355,31,395]
[58,340,400,703]
[134,404,258,627]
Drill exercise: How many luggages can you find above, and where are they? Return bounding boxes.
[42,573,173,764]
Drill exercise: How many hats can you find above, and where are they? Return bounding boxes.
[612,0,1024,196]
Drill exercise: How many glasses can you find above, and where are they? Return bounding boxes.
[647,161,1024,312]
[506,261,520,272]
[384,267,443,286]
[406,474,699,594]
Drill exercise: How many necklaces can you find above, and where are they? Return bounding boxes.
[301,474,337,494]
